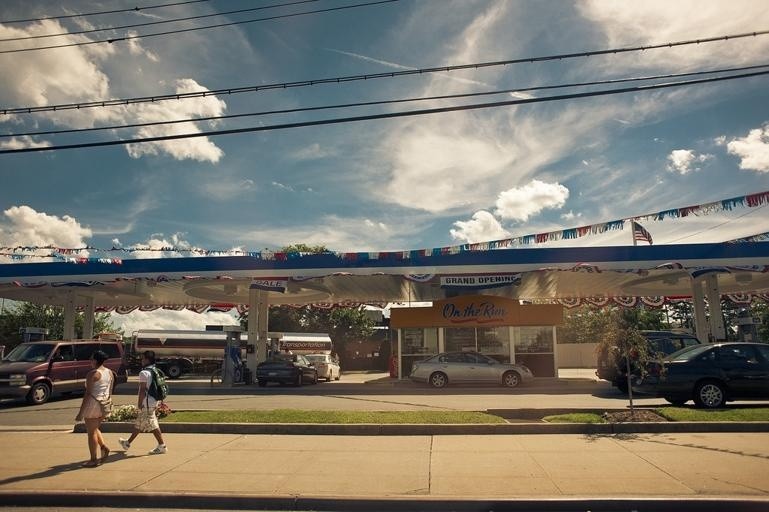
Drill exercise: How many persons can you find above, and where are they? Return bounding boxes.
[75,350,115,467]
[389,352,398,377]
[118,351,168,454]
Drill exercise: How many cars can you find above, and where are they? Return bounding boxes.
[304,354,341,382]
[654,341,769,409]
[255,354,322,387]
[408,350,534,388]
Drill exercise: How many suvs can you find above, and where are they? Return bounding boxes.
[0,338,128,405]
[595,330,702,398]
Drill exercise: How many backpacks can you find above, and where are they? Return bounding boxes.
[144,367,169,400]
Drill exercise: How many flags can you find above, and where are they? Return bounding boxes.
[632,221,653,245]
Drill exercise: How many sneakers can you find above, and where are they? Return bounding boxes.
[119,438,130,450]
[81,460,98,468]
[148,446,168,455]
[99,446,110,463]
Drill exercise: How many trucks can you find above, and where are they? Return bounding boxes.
[93,329,332,379]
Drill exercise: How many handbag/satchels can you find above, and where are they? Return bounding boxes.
[100,397,115,414]
